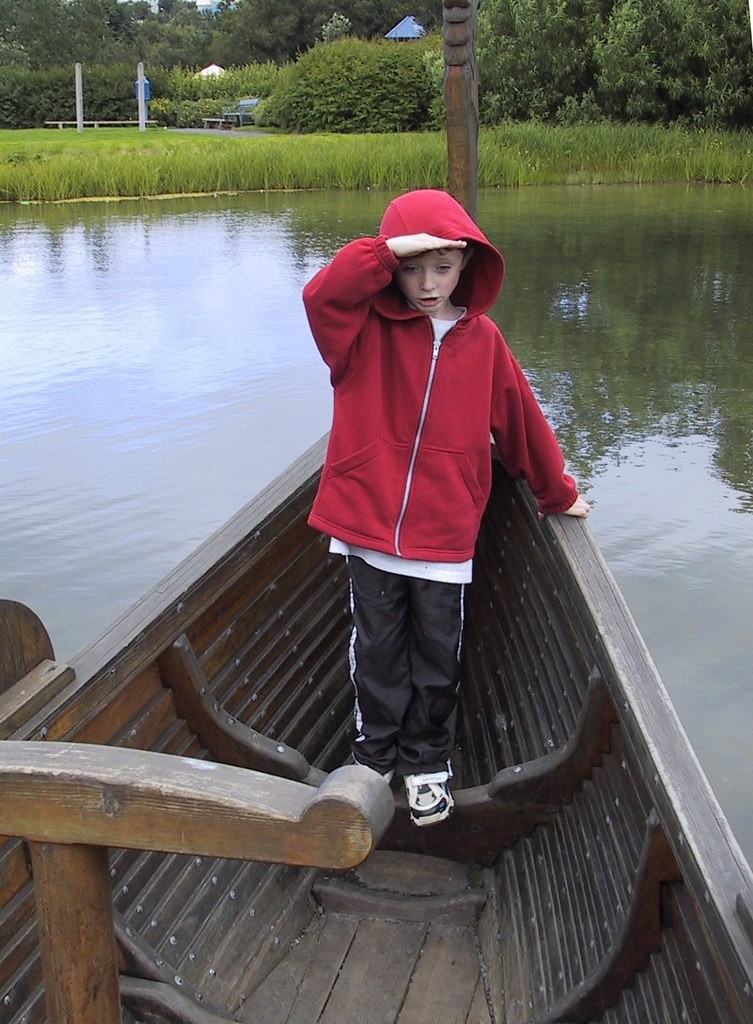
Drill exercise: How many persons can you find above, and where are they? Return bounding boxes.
[300,190,591,829]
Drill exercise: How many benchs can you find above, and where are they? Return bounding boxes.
[201,118,234,130]
[44,121,159,129]
[222,98,260,126]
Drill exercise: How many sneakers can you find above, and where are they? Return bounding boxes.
[350,760,401,794]
[405,759,457,826]
